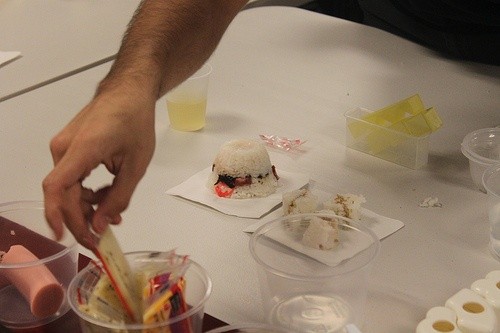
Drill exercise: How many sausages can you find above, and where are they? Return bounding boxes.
[0,245,64,319]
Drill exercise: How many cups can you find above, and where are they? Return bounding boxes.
[481,165,500,260]
[165,65,213,132]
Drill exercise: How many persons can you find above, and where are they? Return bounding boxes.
[41,0,500,249]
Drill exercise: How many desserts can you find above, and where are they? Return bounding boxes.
[348,94,442,151]
[281,188,363,251]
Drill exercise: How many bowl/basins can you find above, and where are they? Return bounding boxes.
[67,248,213,332]
[206,324,307,333]
[0,201,78,327]
[461,126,500,193]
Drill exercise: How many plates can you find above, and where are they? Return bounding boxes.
[165,165,309,218]
[243,208,405,266]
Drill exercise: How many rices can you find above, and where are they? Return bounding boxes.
[207,137,278,199]
